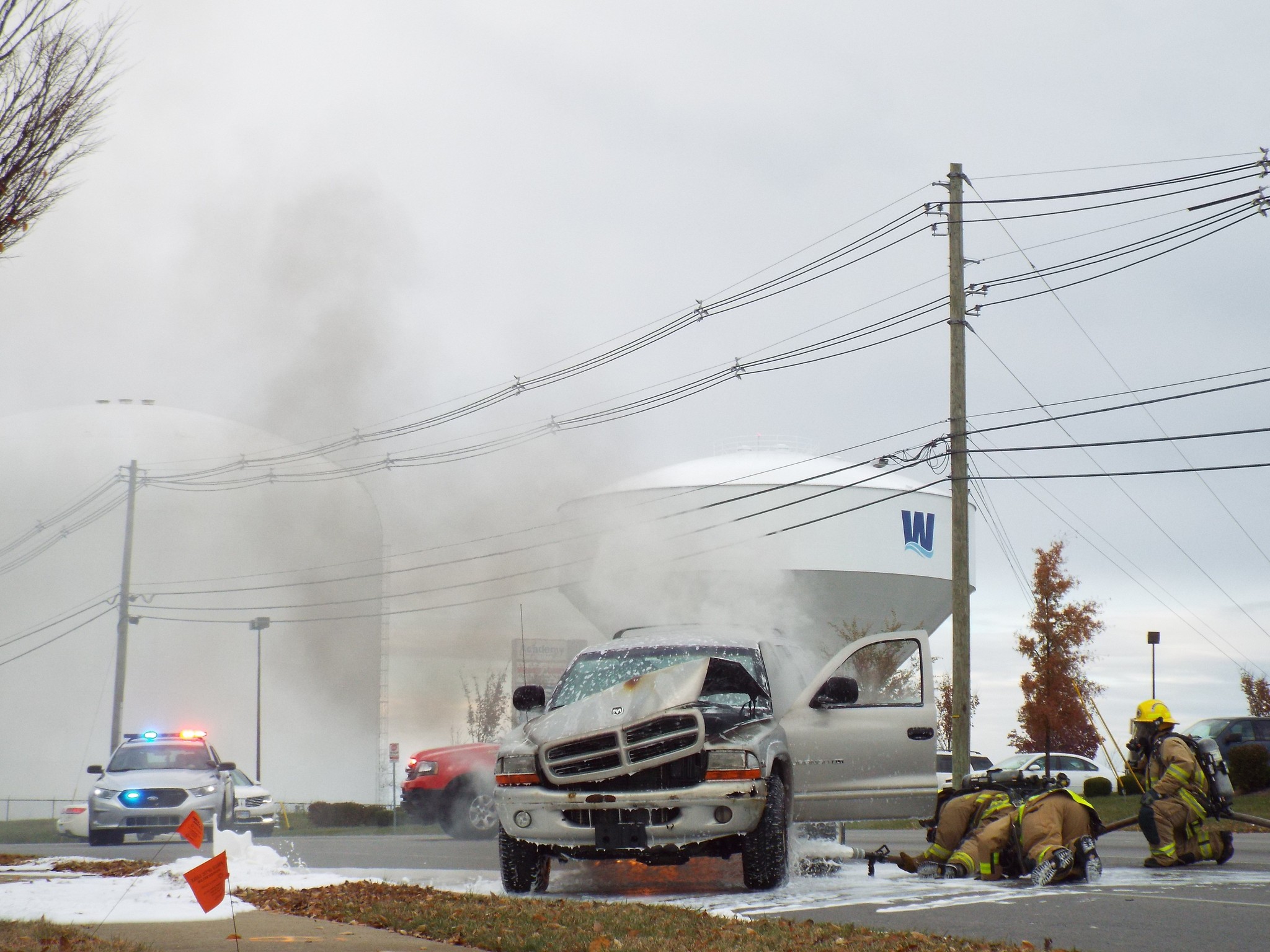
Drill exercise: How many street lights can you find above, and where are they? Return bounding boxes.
[248,617,271,782]
[1148,631,1160,700]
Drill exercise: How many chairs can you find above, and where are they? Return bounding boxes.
[175,754,195,769]
[131,753,148,767]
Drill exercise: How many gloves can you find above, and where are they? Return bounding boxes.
[1140,788,1165,805]
[889,851,919,873]
[927,827,937,842]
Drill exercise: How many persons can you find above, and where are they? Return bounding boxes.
[1127,697,1235,868]
[945,785,1105,885]
[901,777,1024,878]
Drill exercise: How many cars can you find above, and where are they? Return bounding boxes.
[87,730,236,846]
[56,804,90,842]
[229,768,276,838]
[971,752,1126,796]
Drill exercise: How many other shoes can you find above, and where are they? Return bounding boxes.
[1216,831,1234,865]
[1031,849,1074,886]
[917,861,966,879]
[1074,835,1103,882]
[1144,858,1180,867]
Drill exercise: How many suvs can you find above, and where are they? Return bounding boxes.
[492,626,939,896]
[935,748,995,790]
[1179,717,1270,791]
[400,742,501,841]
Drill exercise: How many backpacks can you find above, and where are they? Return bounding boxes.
[1126,736,1149,753]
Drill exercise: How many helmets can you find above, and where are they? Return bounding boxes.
[1132,700,1180,725]
